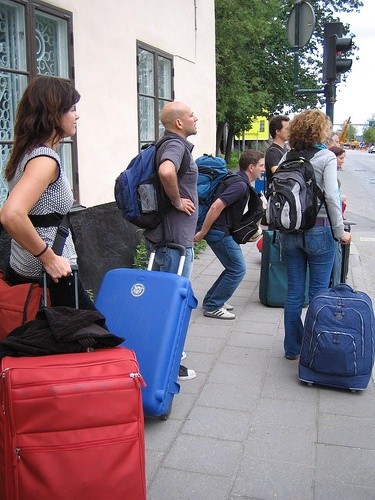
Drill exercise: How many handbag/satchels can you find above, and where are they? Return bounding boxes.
[1,272,52,338]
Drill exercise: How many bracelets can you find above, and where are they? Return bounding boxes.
[34,243,48,257]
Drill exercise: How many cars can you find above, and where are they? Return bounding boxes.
[368,146,375,153]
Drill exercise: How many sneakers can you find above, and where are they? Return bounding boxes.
[221,304,233,311]
[177,365,196,380]
[204,309,235,319]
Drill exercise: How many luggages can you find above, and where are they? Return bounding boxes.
[298,240,374,393]
[95,242,197,422]
[261,226,311,309]
[0,264,147,500]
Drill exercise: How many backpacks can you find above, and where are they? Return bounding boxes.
[114,137,189,229]
[214,177,268,245]
[194,153,235,234]
[269,148,324,233]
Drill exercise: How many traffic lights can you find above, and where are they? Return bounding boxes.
[325,32,352,81]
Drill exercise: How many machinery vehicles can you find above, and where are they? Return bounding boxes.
[339,116,365,151]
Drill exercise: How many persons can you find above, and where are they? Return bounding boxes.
[0,76,95,316]
[193,149,265,319]
[325,131,340,149]
[328,146,347,214]
[265,115,290,191]
[143,102,198,380]
[276,110,351,360]
